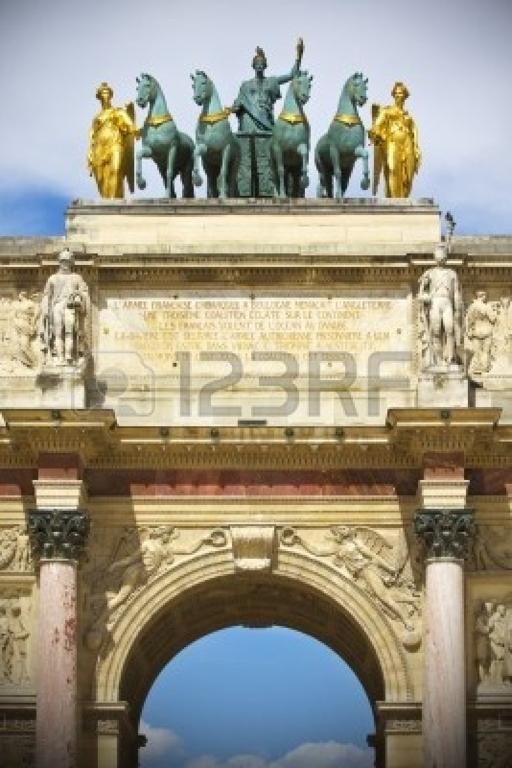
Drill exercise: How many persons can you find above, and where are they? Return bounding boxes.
[86,526,213,649]
[0,606,31,685]
[293,525,421,631]
[87,83,143,198]
[417,250,459,365]
[466,291,497,372]
[475,602,512,685]
[41,252,84,362]
[13,292,40,366]
[227,37,304,131]
[370,84,422,198]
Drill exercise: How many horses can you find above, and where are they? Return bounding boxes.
[189,69,242,199]
[263,66,314,199]
[314,72,370,199]
[135,71,195,198]
